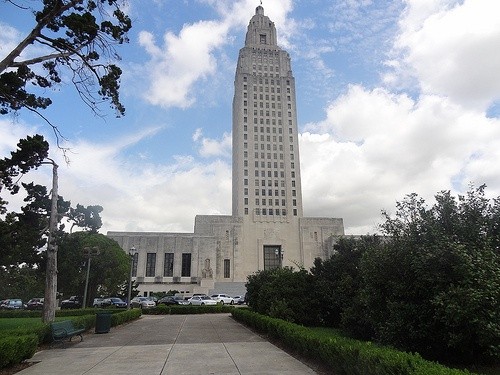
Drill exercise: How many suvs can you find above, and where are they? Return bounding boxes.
[60,295,84,309]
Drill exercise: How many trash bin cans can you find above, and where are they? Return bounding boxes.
[95,311,111,334]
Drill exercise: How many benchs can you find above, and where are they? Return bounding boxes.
[51,320,86,348]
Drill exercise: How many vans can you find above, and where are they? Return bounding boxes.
[0,299,24,310]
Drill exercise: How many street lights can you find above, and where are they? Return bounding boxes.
[82,245,101,308]
[274,248,285,267]
[127,246,136,308]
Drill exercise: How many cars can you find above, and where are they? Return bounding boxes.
[187,295,219,307]
[130,296,191,309]
[211,294,246,305]
[93,297,128,309]
[24,297,45,310]
